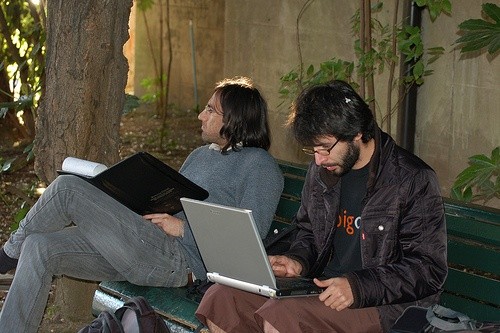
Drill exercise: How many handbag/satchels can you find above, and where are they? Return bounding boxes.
[390,302,472,333]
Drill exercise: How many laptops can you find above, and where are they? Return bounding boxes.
[180,196,329,301]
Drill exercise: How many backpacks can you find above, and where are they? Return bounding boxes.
[78,296,171,333]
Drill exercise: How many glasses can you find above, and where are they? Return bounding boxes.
[300,137,344,156]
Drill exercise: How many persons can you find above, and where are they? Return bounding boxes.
[0,83,284,333]
[194,79,448,333]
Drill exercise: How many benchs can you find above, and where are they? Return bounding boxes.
[91,156,500,333]
